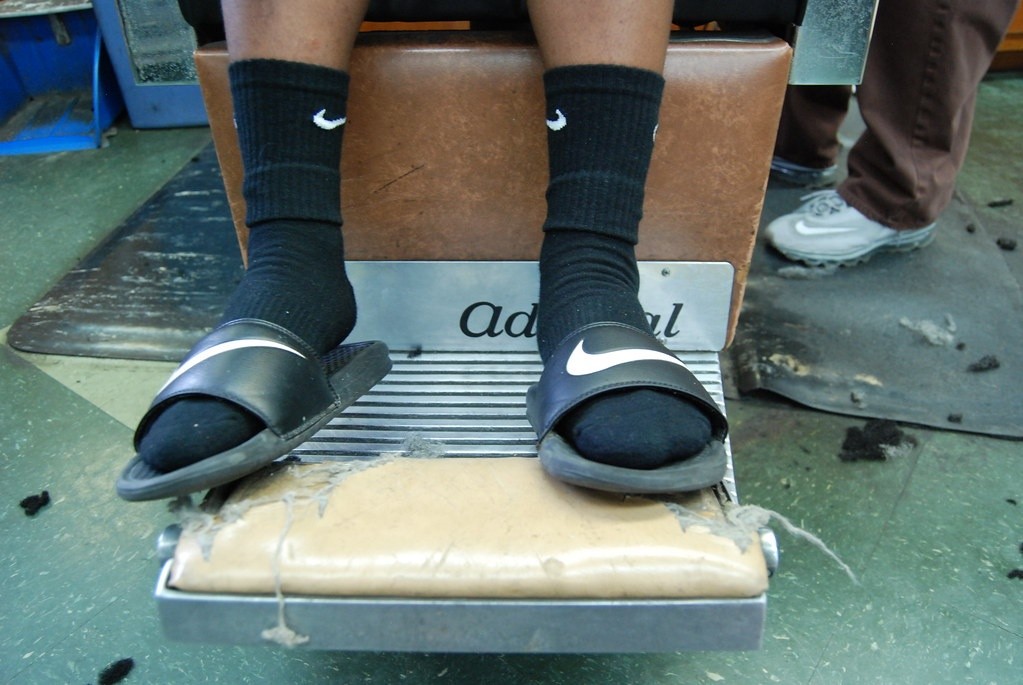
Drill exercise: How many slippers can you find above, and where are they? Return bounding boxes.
[115,316,393,503]
[525,321,728,494]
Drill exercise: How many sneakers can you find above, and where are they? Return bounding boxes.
[770,153,839,189]
[765,188,937,267]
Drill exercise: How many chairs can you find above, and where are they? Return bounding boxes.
[152,33,794,647]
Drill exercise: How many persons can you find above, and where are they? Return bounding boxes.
[116,0,729,501]
[766,0,1016,267]
